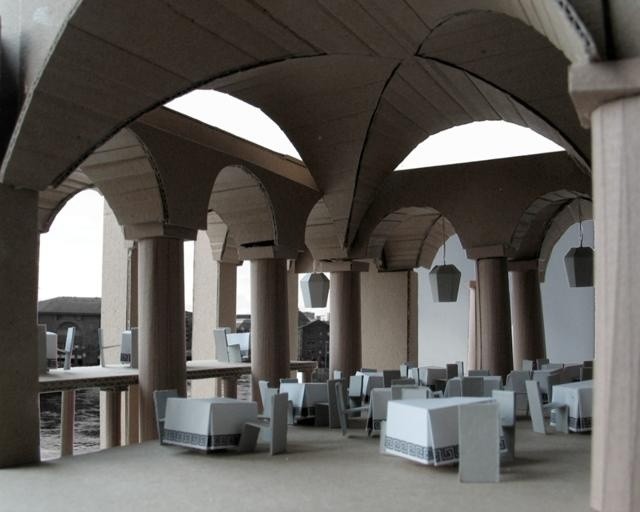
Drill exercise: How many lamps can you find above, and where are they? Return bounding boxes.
[299,273,330,309]
[563,196,595,288]
[427,216,462,303]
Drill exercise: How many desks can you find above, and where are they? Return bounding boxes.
[45,331,57,367]
[225,333,250,361]
[119,330,131,363]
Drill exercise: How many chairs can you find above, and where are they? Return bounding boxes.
[212,328,243,363]
[97,327,122,367]
[221,327,232,333]
[38,323,49,374]
[57,326,76,370]
